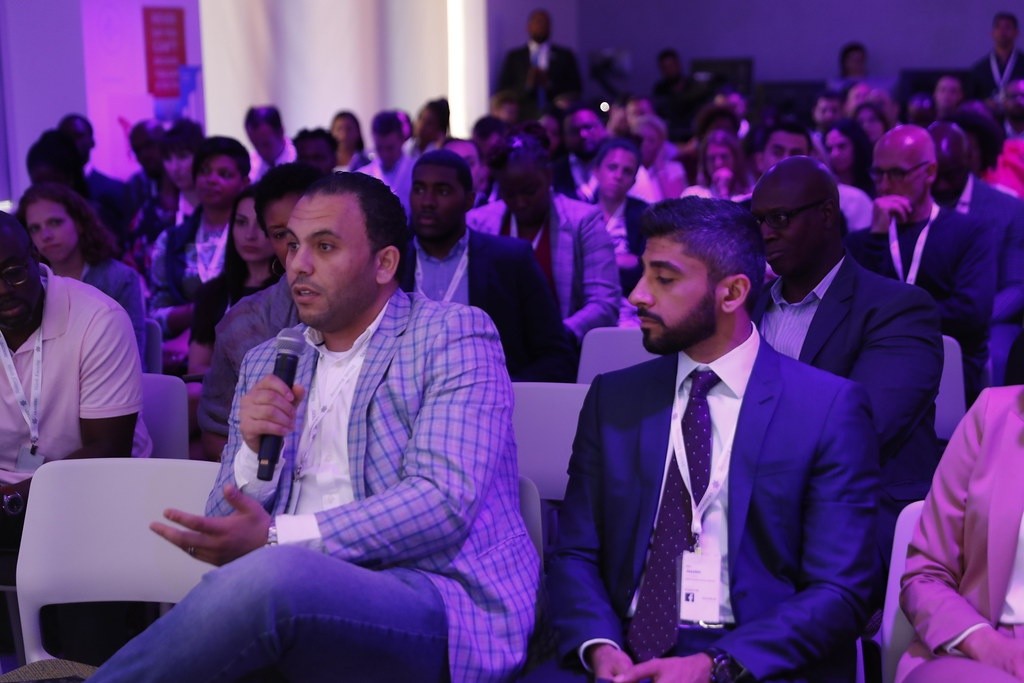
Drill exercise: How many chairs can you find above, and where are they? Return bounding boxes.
[510,328,661,589]
[881,500,924,683]
[934,334,967,441]
[17,319,222,664]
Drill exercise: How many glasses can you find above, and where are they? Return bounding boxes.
[868,161,928,181]
[753,198,835,229]
[0,247,33,288]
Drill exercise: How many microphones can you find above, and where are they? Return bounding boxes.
[256,327,306,481]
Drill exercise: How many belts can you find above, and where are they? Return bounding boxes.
[679,620,736,629]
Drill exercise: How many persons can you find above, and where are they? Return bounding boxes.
[0,6,1023,682]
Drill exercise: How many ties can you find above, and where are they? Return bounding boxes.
[627,369,721,662]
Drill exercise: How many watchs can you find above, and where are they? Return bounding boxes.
[702,646,736,683]
[263,515,277,546]
